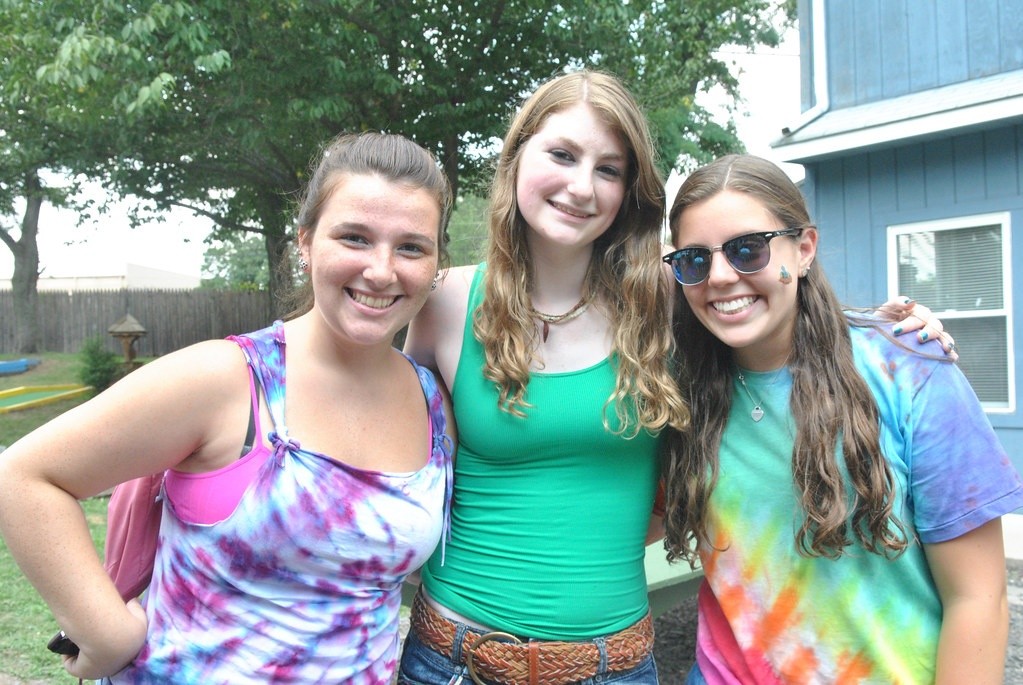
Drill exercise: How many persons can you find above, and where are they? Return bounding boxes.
[645,155,1022,685]
[401,65,957,685]
[0,133,456,685]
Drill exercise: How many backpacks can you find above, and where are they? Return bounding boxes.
[101,466,164,606]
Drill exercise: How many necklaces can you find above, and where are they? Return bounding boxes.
[733,344,797,422]
[525,272,601,340]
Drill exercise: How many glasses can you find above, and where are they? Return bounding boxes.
[662,228,803,287]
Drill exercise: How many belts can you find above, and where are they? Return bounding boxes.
[408,585,655,685]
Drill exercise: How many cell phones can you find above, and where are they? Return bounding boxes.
[47,630,81,656]
